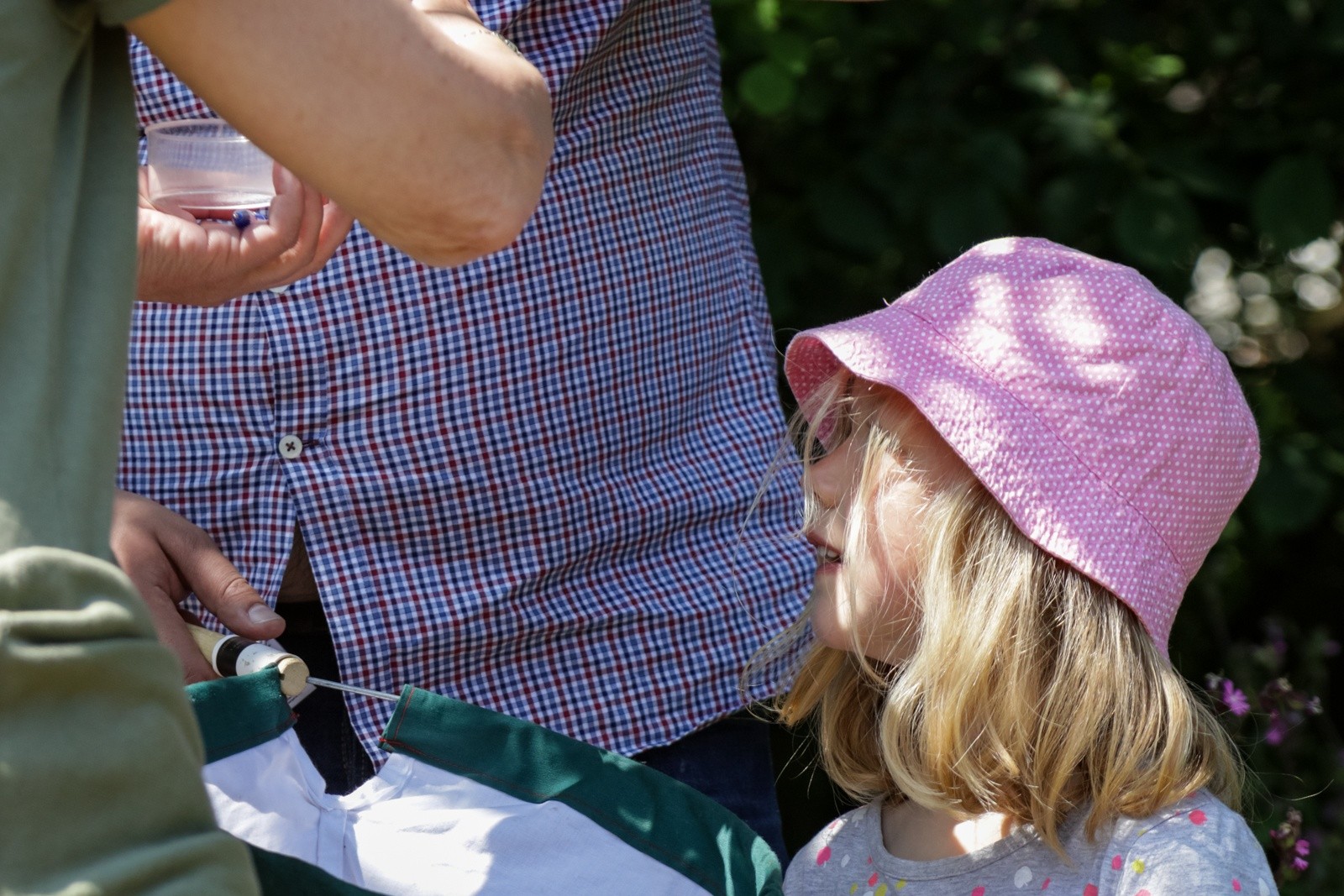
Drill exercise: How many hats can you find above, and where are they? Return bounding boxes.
[784,236,1262,660]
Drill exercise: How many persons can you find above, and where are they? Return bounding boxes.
[0,0,554,896]
[782,233,1277,896]
[118,0,856,896]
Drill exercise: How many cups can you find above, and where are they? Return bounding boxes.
[143,117,275,210]
